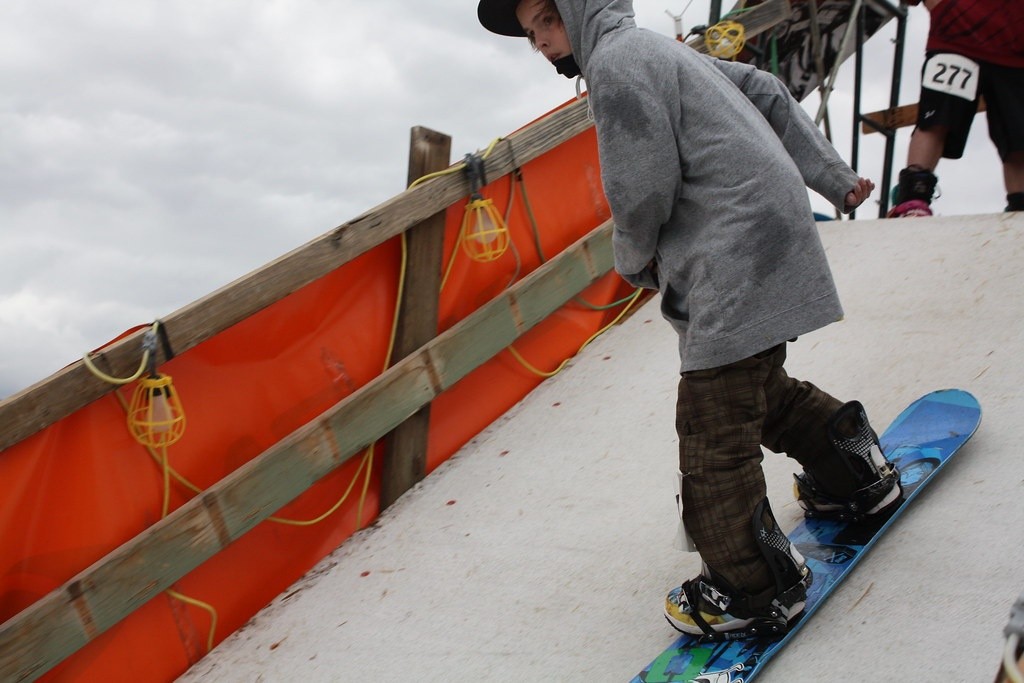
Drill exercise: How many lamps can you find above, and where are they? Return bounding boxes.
[692,23,746,57]
[126,333,186,446]
[460,160,510,261]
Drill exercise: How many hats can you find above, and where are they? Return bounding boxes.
[477,0,528,37]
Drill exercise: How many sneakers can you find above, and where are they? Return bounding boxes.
[664,497,813,637]
[792,401,902,519]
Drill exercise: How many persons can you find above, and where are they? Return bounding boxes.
[886,0,1024,218]
[478,0,903,644]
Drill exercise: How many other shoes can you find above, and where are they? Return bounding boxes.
[887,182,934,218]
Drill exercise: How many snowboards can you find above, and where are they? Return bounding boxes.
[628,385,987,683]
